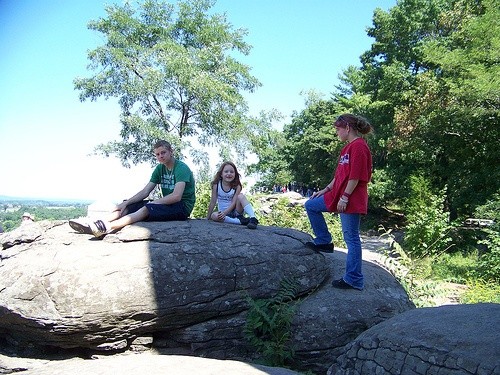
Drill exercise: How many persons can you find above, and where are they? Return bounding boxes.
[21,212,33,226]
[69,140,195,238]
[251,181,319,198]
[305,114,372,290]
[196,161,259,229]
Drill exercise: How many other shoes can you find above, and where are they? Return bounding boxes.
[247,216,259,229]
[88,219,115,237]
[306,241,334,253]
[69,218,93,234]
[237,215,251,225]
[332,278,363,290]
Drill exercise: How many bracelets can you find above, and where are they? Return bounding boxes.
[343,192,350,197]
[326,186,330,192]
[340,198,349,203]
